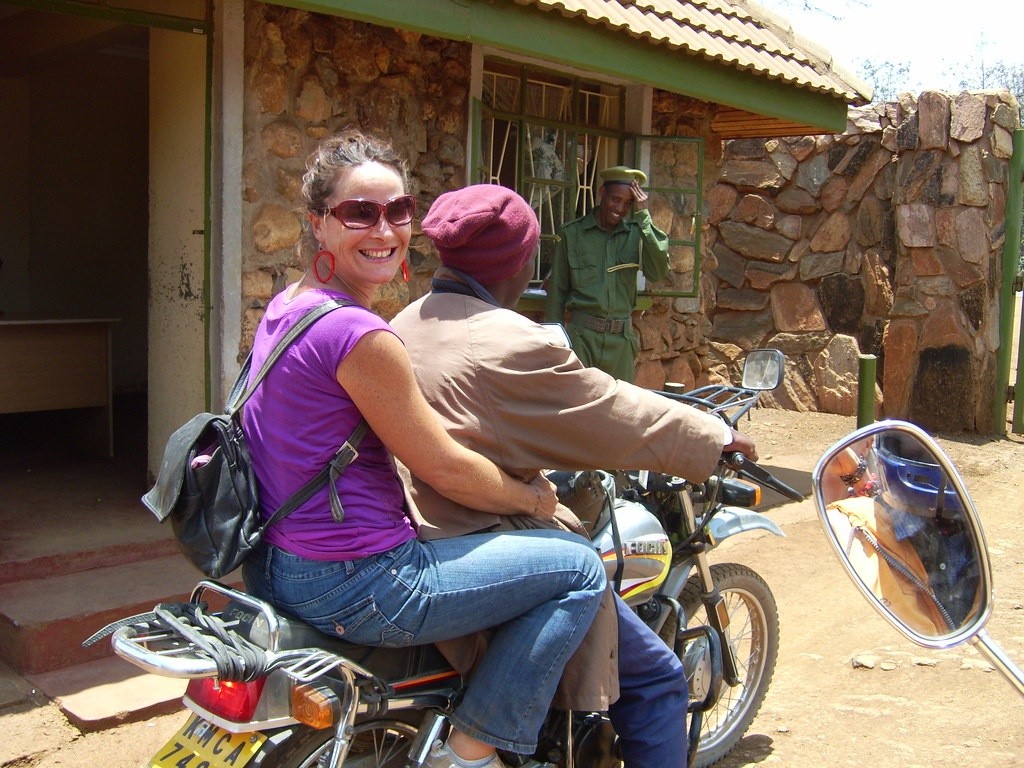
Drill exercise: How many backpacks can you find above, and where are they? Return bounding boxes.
[142,299,370,579]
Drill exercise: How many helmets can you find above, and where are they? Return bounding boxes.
[876,428,962,520]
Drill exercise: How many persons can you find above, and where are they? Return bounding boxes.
[241,130,608,768]
[821,433,873,506]
[546,165,669,382]
[387,184,760,768]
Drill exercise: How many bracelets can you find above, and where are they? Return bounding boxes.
[531,485,540,518]
[840,459,869,487]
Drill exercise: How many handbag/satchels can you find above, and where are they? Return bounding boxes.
[545,469,616,538]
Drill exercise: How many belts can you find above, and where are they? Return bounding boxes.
[585,315,624,333]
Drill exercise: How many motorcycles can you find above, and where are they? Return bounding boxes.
[115,346,807,768]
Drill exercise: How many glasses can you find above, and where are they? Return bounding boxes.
[308,195,416,229]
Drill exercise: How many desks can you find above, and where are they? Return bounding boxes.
[0,313,124,459]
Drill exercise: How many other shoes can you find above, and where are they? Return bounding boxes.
[418,739,507,768]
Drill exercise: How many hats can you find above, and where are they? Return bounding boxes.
[600,166,647,186]
[420,184,539,287]
